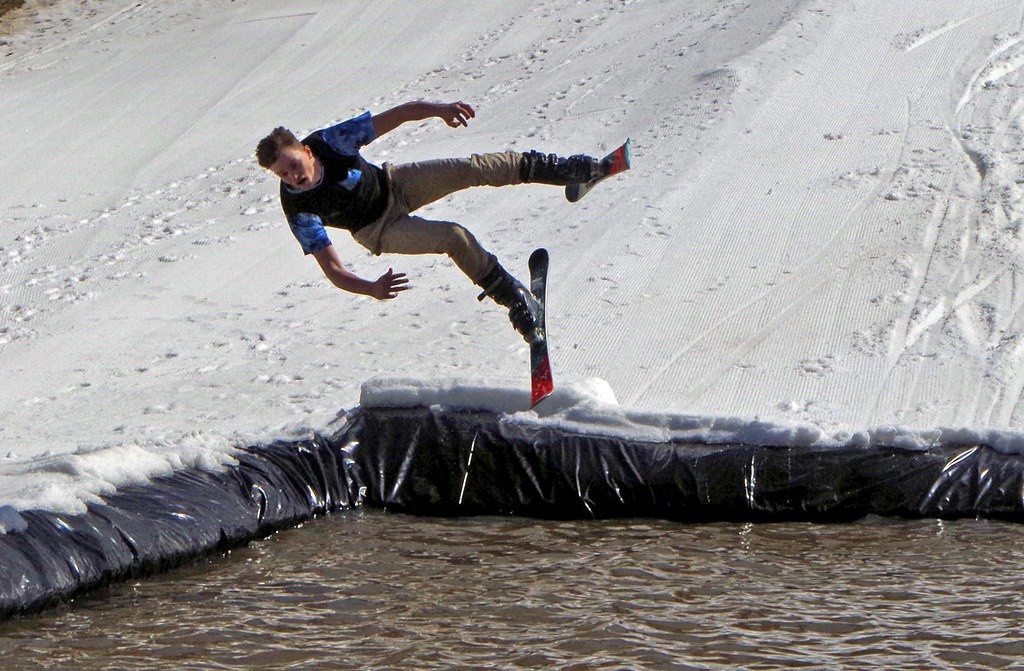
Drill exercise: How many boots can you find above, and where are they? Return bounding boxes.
[520,149,601,186]
[476,262,544,336]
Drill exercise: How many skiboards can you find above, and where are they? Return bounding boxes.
[524,142,632,414]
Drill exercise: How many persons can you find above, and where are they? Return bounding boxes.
[256,101,613,347]
